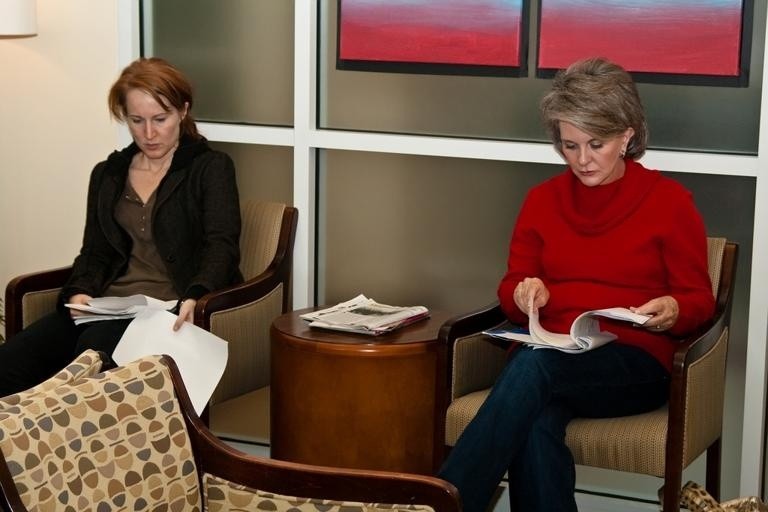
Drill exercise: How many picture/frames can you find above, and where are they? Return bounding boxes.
[532,1,756,91]
[335,0,532,84]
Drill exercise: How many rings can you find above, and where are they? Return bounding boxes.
[656,325,660,329]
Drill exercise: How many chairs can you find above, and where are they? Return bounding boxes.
[428,232,742,512]
[2,349,457,512]
[0,347,116,423]
[7,197,301,430]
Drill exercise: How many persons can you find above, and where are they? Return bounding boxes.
[0,56,245,398]
[436,55,716,511]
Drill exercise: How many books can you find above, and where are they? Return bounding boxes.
[62,293,180,324]
[482,292,654,355]
[299,293,431,338]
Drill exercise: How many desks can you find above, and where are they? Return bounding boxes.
[264,304,459,478]
